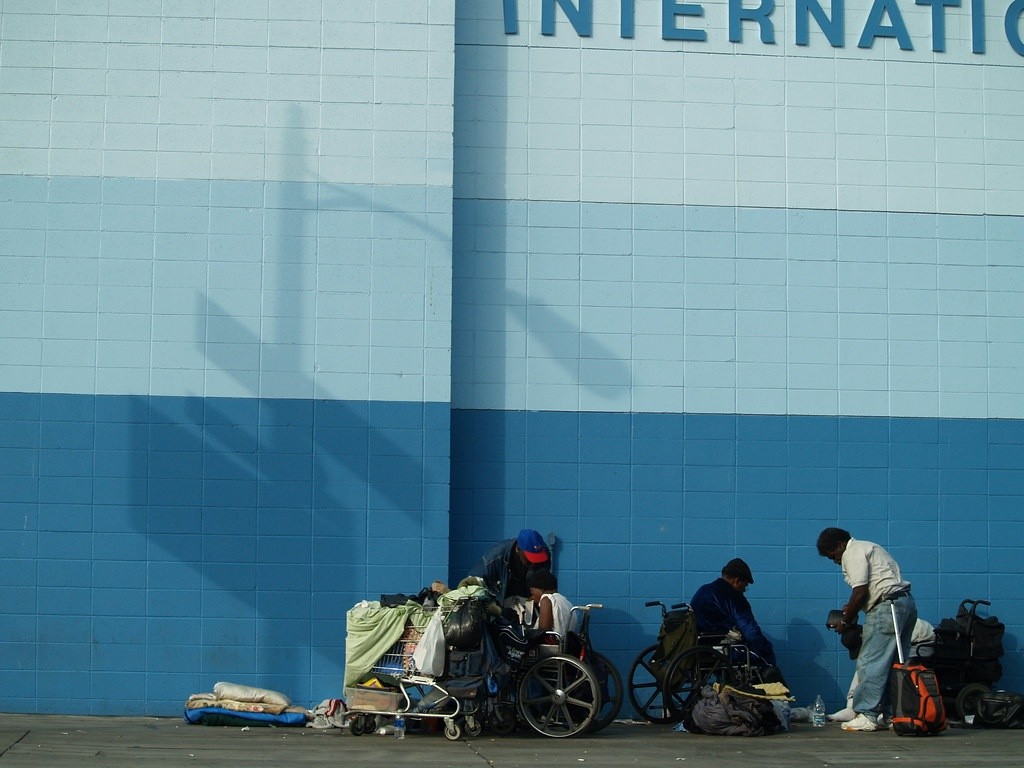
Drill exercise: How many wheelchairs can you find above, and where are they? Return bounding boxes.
[627,600,763,734]
[915,600,993,728]
[498,603,623,739]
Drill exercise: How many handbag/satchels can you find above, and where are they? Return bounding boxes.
[412,607,444,676]
[444,602,484,650]
[974,690,1024,730]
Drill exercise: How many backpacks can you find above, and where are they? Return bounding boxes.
[889,661,948,737]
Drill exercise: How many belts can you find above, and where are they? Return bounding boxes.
[869,590,912,612]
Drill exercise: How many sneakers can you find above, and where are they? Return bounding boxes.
[826,706,856,721]
[840,714,878,732]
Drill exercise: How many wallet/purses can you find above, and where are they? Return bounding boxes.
[826,609,858,628]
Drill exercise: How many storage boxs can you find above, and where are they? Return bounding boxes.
[344,687,404,713]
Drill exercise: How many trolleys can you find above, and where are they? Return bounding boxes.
[342,596,486,739]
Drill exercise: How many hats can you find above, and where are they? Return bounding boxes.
[727,558,753,584]
[517,529,548,562]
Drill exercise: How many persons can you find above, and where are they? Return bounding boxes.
[528,570,578,642]
[469,529,551,603]
[691,558,784,685]
[826,619,936,722]
[817,528,916,732]
